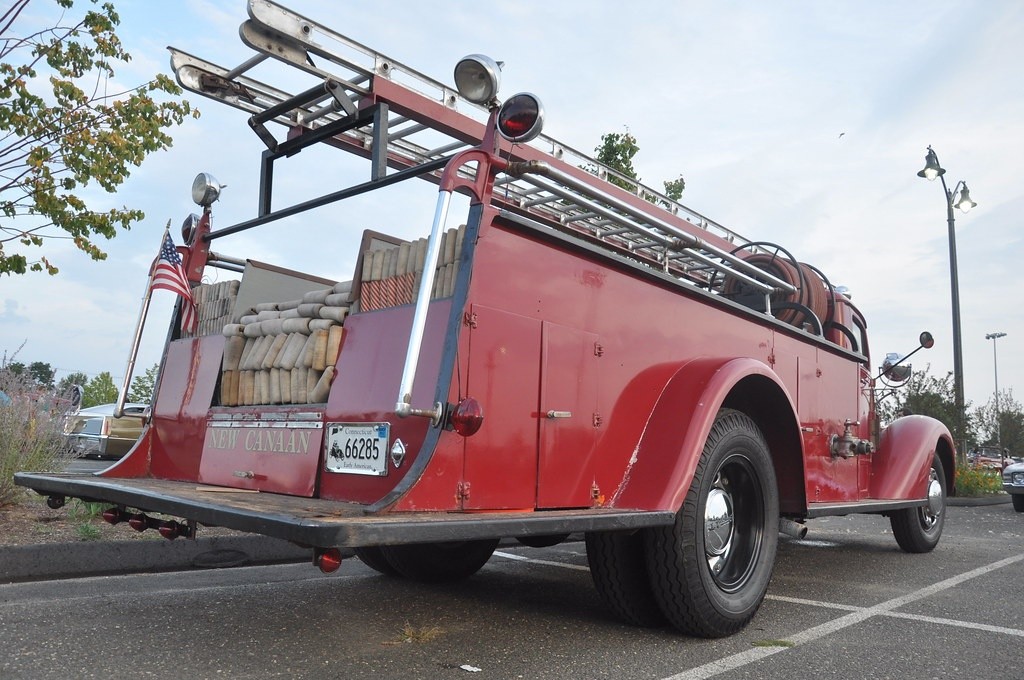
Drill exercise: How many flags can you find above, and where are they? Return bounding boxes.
[152,232,198,335]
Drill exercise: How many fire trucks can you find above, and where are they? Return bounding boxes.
[13,1,958,640]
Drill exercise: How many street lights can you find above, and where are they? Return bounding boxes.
[914,143,978,466]
[985,332,1007,444]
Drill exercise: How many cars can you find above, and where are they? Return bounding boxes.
[59,400,152,459]
[967,444,1024,514]
